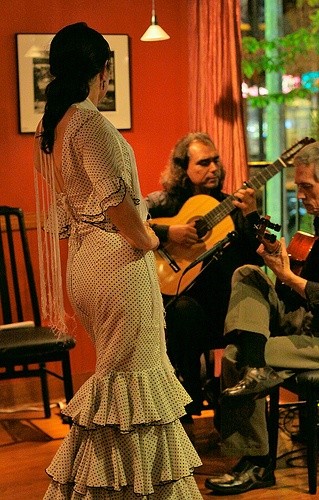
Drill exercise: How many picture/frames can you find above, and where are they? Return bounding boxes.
[16,31,131,134]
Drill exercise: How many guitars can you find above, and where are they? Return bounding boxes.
[253,214,318,311]
[146,135,317,296]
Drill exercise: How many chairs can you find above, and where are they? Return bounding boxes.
[264,230,319,495]
[0,206,77,418]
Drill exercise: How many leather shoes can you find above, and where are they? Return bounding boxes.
[204,457,276,495]
[222,364,284,397]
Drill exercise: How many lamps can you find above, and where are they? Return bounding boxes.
[141,0,170,41]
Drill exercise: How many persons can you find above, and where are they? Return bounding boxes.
[202,141,319,495]
[35,21,207,500]
[146,133,256,436]
